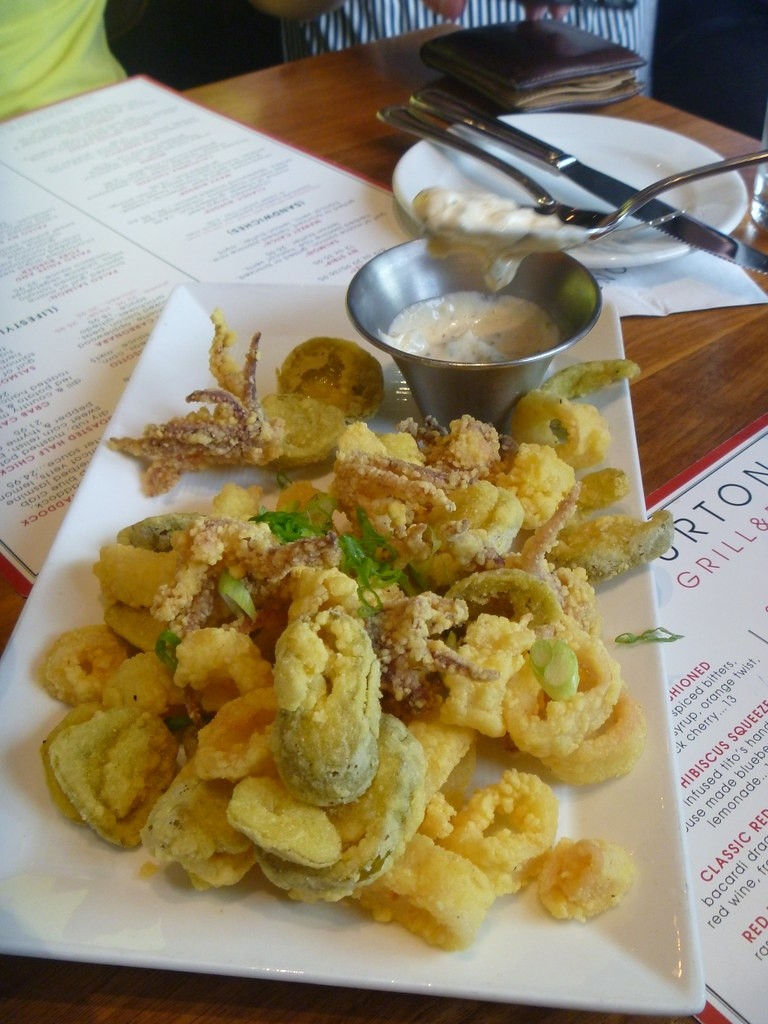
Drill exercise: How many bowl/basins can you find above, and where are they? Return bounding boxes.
[343,232,602,430]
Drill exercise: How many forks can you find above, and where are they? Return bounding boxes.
[374,102,686,238]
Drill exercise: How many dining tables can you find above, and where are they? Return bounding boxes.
[1,22,768,1024]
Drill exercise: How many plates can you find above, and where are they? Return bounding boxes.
[1,280,709,1018]
[393,112,747,270]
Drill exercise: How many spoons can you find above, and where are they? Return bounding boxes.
[519,146,768,249]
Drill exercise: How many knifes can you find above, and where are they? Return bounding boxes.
[410,87,768,276]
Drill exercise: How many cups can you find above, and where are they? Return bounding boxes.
[749,105,768,234]
